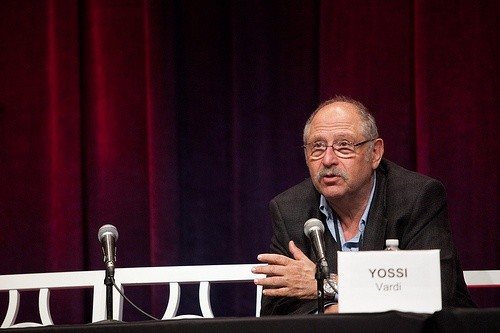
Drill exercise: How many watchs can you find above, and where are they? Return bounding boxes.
[323,273,337,299]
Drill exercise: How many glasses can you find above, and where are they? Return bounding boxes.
[303,139,376,160]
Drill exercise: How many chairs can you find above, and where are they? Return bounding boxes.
[0,270,110,328]
[112,263,268,323]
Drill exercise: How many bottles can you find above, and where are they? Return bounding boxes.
[384,239,400,250]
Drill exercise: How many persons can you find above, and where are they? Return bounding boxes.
[251,96,477,316]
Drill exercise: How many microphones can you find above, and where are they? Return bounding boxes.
[98,224,118,280]
[303,218,330,279]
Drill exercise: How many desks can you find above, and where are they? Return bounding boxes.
[0,308,500,333]
